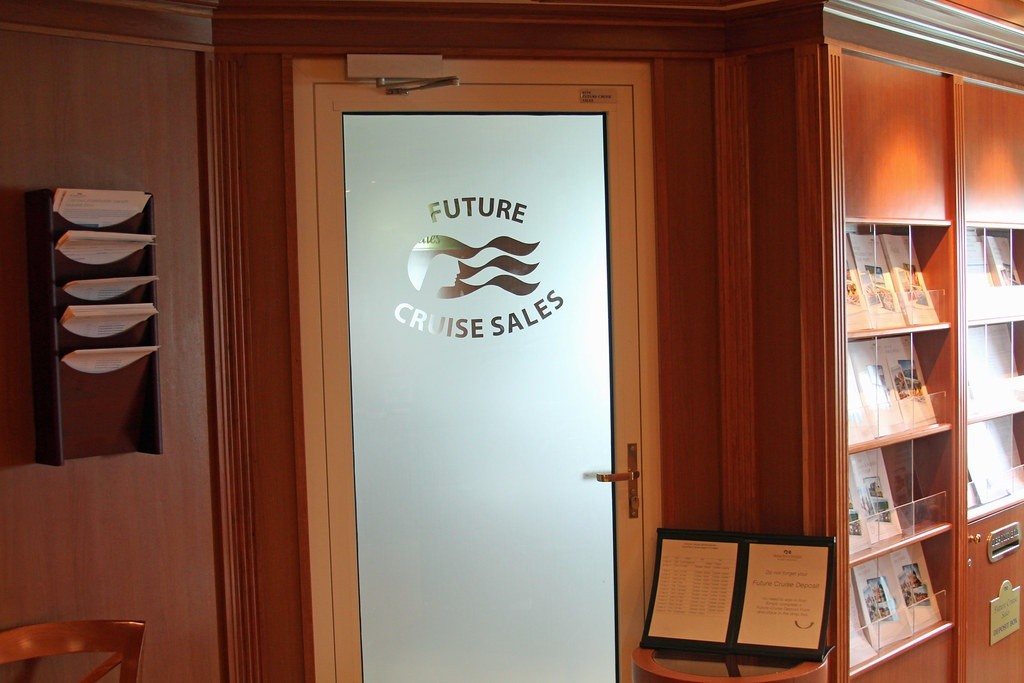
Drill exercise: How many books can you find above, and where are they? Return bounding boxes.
[968,414,1023,510]
[849,450,903,553]
[836,338,947,446]
[965,227,1022,289]
[850,542,943,669]
[842,227,941,334]
[969,323,1019,386]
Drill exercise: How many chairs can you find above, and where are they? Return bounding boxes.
[1,614,148,683]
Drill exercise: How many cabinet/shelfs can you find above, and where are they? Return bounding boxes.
[23,187,165,465]
[727,36,1023,681]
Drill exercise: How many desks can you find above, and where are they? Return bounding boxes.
[632,644,830,683]
